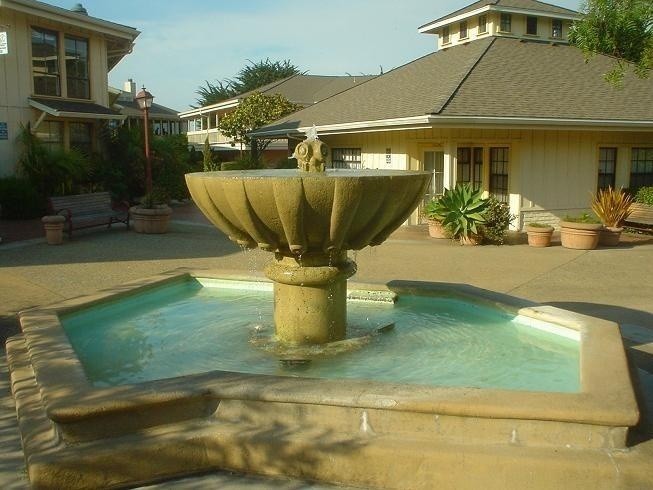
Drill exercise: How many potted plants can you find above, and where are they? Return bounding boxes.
[128,190,173,234]
[526,221,554,247]
[591,185,635,246]
[420,182,516,246]
[559,210,603,250]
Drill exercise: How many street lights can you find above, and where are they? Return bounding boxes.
[135,85,152,204]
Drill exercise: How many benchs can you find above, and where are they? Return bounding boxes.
[34,192,129,240]
[623,203,653,234]
